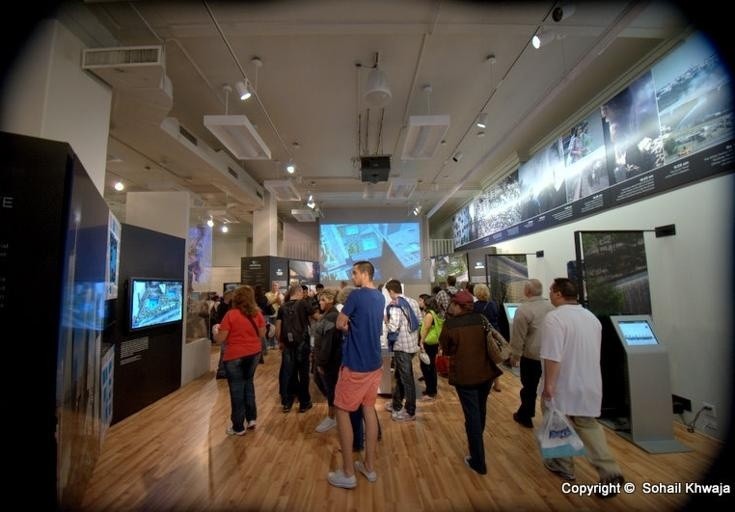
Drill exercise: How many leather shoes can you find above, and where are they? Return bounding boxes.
[299,402,313,413]
[283,405,290,412]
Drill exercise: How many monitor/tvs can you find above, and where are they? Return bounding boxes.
[129,277,186,328]
[618,319,660,347]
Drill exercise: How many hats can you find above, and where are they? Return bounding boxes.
[451,293,473,304]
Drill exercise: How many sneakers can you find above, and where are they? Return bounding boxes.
[354,460,377,482]
[464,456,487,476]
[514,413,534,428]
[594,474,624,500]
[422,391,437,395]
[247,420,257,430]
[225,426,245,436]
[543,458,576,482]
[391,410,415,422]
[384,402,403,412]
[327,472,357,489]
[315,416,337,432]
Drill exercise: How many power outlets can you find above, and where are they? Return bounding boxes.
[700,402,716,418]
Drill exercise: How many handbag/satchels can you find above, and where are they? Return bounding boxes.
[419,310,446,345]
[435,349,451,374]
[481,315,513,364]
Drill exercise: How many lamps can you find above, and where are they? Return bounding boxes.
[306,194,316,209]
[284,158,297,175]
[532,25,566,49]
[200,214,217,229]
[476,113,489,130]
[415,201,424,214]
[363,62,393,111]
[234,77,252,102]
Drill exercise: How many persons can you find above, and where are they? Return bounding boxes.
[418,297,445,401]
[438,291,503,474]
[276,285,319,412]
[567,124,588,203]
[539,278,624,497]
[336,286,382,463]
[256,285,272,356]
[266,282,282,350]
[432,286,441,296]
[417,294,429,318]
[301,285,308,300]
[511,280,554,427]
[465,281,479,302]
[212,285,267,436]
[312,290,343,433]
[314,284,323,299]
[436,275,457,314]
[208,295,219,343]
[474,283,501,392]
[327,260,386,489]
[383,280,422,421]
[216,290,232,379]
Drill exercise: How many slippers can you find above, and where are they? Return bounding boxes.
[493,386,501,392]
